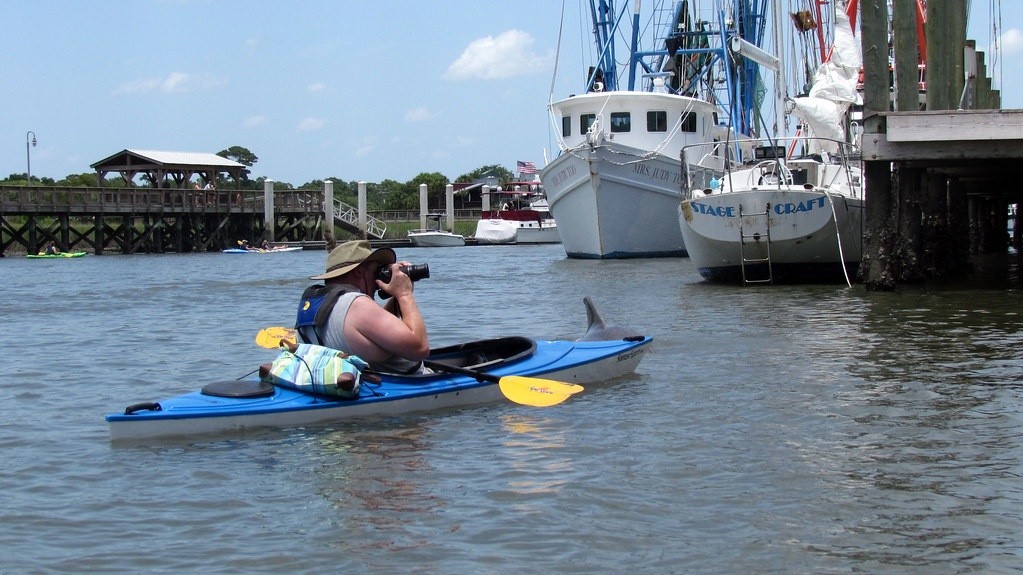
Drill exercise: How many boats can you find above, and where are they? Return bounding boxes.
[106,294,654,443]
[406,228,466,248]
[474,178,563,245]
[26,250,86,258]
[221,245,303,255]
[528,1,925,285]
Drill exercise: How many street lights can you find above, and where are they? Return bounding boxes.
[25,129,37,199]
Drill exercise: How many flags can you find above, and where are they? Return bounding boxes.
[517,161,536,174]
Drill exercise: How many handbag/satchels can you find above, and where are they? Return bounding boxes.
[259,339,382,399]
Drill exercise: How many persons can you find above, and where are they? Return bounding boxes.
[194,182,201,207]
[240,240,259,250]
[508,201,517,211]
[204,180,216,206]
[503,202,509,211]
[46,241,60,255]
[234,192,241,205]
[261,239,288,250]
[294,240,435,376]
[497,201,502,216]
[507,185,512,198]
[502,184,506,197]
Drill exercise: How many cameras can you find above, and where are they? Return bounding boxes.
[375,262,429,299]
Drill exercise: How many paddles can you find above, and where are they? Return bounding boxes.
[253,325,586,408]
[39,252,74,258]
[237,240,266,253]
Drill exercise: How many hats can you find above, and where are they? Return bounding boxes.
[308,240,397,280]
